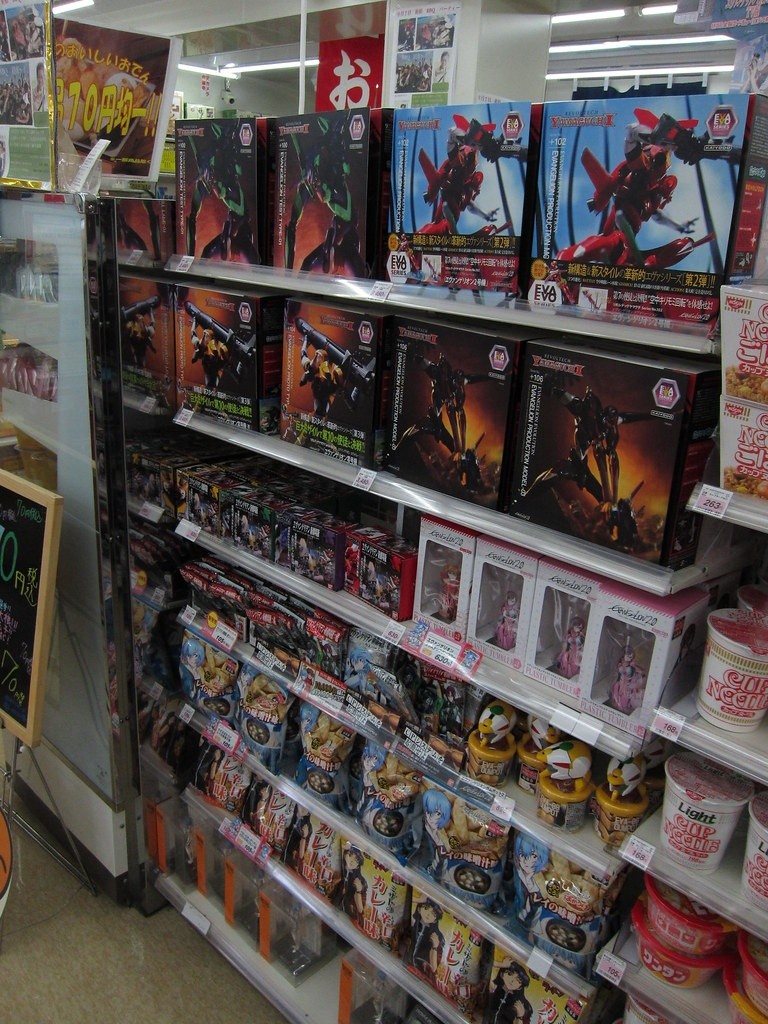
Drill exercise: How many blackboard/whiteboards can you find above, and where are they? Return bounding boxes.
[1,468,65,749]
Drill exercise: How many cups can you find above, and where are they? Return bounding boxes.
[59,152,102,196]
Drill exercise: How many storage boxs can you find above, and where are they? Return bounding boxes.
[154,797,408,1024]
[118,90,768,746]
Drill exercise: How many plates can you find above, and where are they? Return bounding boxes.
[72,58,155,157]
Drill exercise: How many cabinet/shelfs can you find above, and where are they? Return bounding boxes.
[591,479,768,1024]
[145,254,760,1024]
